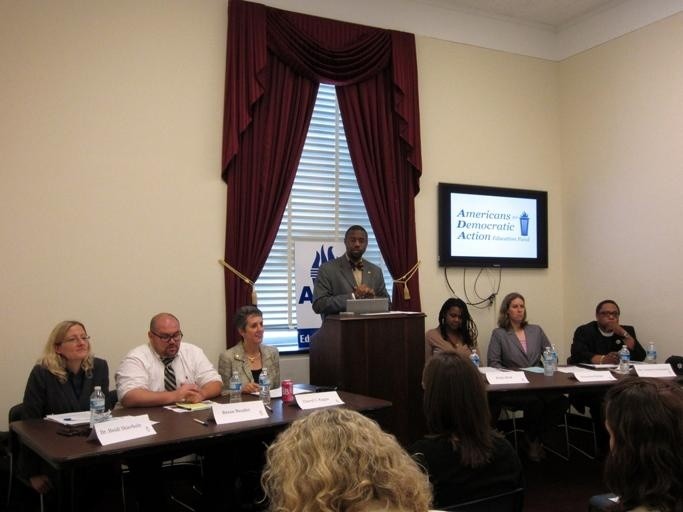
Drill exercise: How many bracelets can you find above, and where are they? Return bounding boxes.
[599,355,605,364]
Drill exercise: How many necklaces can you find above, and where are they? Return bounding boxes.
[454,337,460,348]
[248,352,260,363]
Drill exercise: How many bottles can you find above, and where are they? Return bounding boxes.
[550,343,557,372]
[89,386,104,429]
[470,349,479,366]
[544,347,552,376]
[258,368,270,404]
[618,345,629,373]
[229,371,239,404]
[520,211,529,236]
[646,342,656,364]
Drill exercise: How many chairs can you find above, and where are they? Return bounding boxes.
[109,390,195,512]
[437,486,526,512]
[500,405,571,465]
[4,403,127,512]
[559,356,603,460]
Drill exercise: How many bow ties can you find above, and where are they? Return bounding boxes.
[350,260,363,271]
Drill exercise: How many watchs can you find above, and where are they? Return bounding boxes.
[624,332,629,338]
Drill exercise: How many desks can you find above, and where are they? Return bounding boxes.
[10,380,394,512]
[479,362,683,397]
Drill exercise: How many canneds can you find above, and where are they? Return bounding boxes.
[282,380,293,402]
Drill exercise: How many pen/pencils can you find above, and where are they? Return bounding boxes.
[265,405,272,412]
[193,419,209,426]
[64,417,93,421]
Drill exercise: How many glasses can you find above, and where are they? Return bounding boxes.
[598,310,618,317]
[61,335,90,344]
[150,330,183,342]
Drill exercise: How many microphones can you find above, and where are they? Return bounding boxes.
[352,293,356,300]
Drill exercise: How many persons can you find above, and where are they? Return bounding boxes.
[114,312,226,511]
[404,349,527,512]
[424,297,483,368]
[486,292,569,463]
[16,321,111,512]
[603,373,683,512]
[217,305,281,397]
[256,405,435,512]
[310,223,392,322]
[571,299,647,365]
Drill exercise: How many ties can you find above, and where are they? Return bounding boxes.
[162,358,177,391]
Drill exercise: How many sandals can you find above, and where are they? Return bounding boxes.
[526,439,546,462]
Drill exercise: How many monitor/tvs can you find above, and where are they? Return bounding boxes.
[438,182,549,269]
